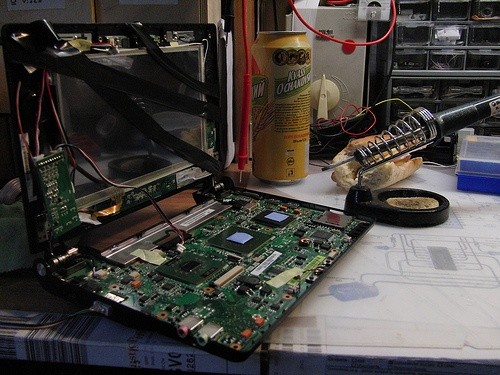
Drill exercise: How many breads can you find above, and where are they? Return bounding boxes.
[331,134,423,192]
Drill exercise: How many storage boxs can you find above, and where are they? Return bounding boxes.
[455,127,500,196]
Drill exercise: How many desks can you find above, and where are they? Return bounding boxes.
[1,157,500,375]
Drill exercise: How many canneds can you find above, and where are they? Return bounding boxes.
[251,30,312,186]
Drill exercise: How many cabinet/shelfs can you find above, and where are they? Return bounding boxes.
[384,0,500,135]
